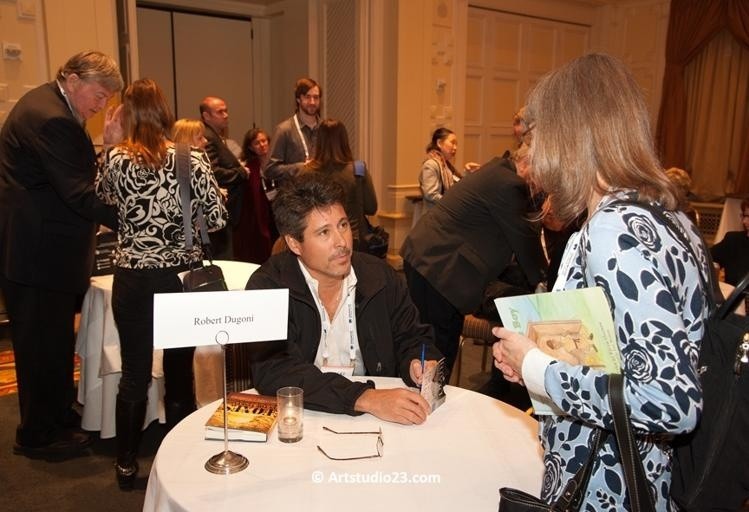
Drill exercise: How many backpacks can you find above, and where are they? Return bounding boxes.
[580,197,749,512]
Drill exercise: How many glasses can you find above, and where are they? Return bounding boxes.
[520,123,536,146]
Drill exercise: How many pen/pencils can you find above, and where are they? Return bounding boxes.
[420,343,425,392]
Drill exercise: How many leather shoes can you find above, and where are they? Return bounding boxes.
[10,413,96,458]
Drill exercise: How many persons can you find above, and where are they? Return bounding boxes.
[262,78,323,177]
[240,128,271,169]
[490,52,711,512]
[400,143,551,385]
[293,118,378,240]
[708,200,749,292]
[417,129,482,218]
[243,170,450,426]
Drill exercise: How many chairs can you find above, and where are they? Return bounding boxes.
[92,230,119,276]
[225,342,254,395]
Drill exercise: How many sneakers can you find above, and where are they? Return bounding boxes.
[115,460,141,494]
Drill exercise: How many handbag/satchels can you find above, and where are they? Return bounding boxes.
[182,263,228,292]
[359,224,390,264]
[497,372,656,512]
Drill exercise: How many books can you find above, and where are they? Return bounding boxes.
[204,392,280,441]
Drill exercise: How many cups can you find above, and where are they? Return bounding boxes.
[275,385,306,444]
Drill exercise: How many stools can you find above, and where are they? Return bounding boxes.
[453,311,499,388]
